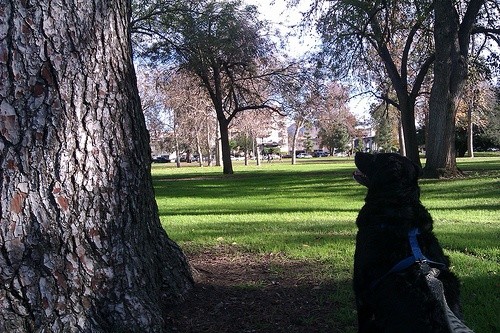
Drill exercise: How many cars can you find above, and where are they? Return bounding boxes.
[312,149,329,157]
[230,155,292,160]
[176,155,214,163]
[152,157,170,163]
[297,153,312,159]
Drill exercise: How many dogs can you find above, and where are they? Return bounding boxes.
[352,150,462,333]
